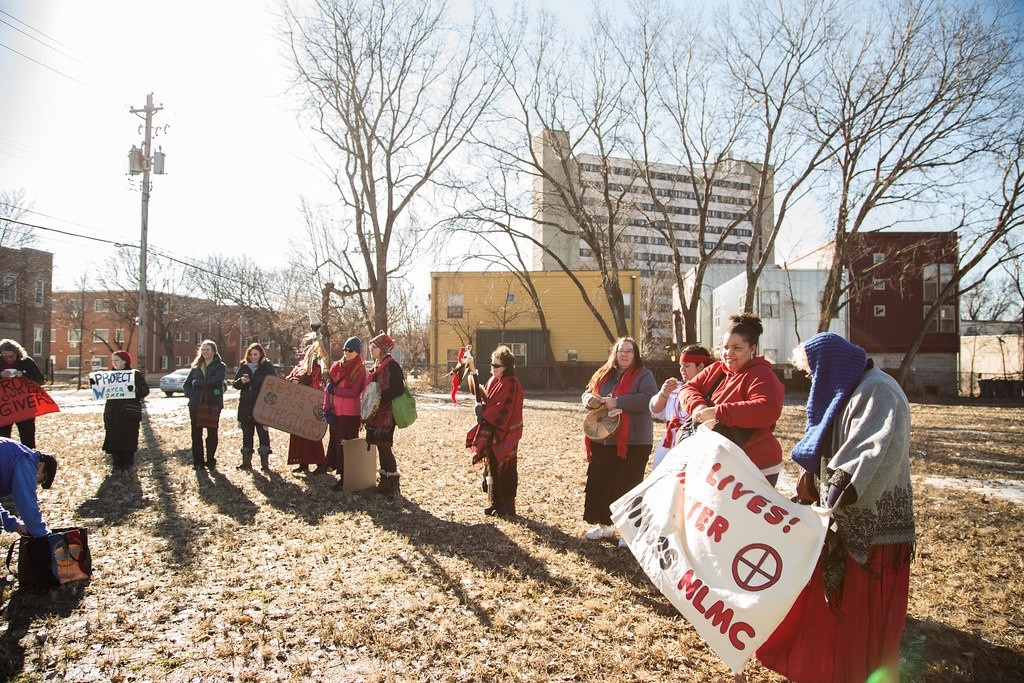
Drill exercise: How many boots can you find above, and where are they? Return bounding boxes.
[236,448,254,470]
[258,448,272,471]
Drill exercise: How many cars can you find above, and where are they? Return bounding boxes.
[160,368,228,397]
[410,367,423,375]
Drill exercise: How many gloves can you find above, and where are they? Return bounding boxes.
[324,383,335,394]
[587,395,603,409]
[324,412,336,424]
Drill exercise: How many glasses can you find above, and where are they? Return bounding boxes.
[616,349,632,354]
[1,353,16,359]
[36,472,46,485]
[342,349,353,353]
[368,345,378,350]
[490,362,504,368]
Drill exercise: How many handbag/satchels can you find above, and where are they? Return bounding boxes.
[195,381,218,428]
[386,362,418,429]
[675,397,755,448]
[7,527,92,593]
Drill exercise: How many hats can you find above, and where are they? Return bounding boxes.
[343,336,360,355]
[112,350,132,364]
[42,453,57,489]
[370,330,395,353]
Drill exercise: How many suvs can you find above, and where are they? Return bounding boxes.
[274,364,294,379]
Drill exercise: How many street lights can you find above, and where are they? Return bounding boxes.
[114,242,147,381]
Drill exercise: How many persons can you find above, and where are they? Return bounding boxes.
[659,390,667,398]
[465,345,524,515]
[283,324,329,477]
[646,344,716,468]
[182,340,226,470]
[756,332,915,683]
[0,338,45,449]
[681,313,785,490]
[90,351,150,471]
[231,342,276,471]
[580,337,659,548]
[323,337,368,488]
[363,332,405,494]
[0,437,57,537]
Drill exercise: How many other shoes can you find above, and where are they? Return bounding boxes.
[618,538,628,548]
[123,460,133,470]
[292,461,400,495]
[485,506,495,515]
[112,463,122,472]
[494,504,516,515]
[586,523,614,539]
